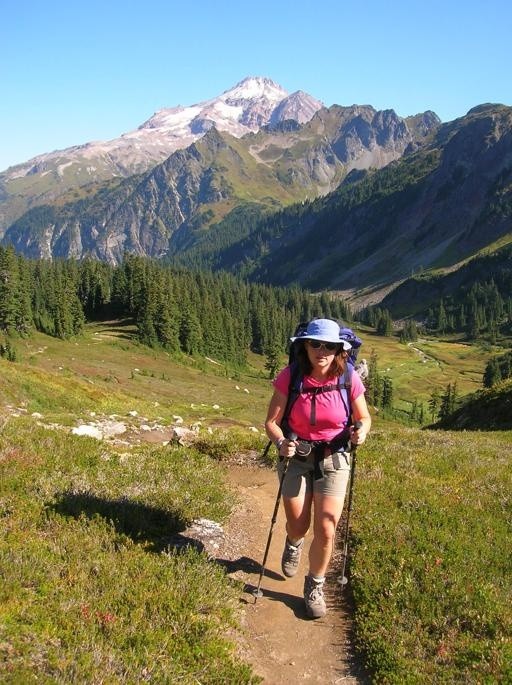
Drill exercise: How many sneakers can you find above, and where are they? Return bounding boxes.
[303,575,327,619]
[281,535,304,579]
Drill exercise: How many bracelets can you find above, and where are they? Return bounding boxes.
[275,438,285,449]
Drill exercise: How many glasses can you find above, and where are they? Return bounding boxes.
[304,340,337,352]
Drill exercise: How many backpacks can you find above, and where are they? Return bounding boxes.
[282,317,362,430]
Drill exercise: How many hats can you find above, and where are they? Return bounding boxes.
[289,319,353,351]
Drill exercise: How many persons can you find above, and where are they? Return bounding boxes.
[264,318,372,620]
